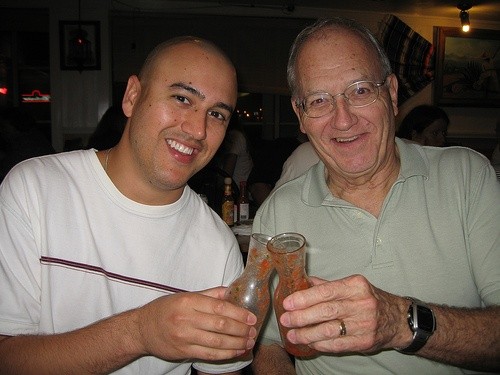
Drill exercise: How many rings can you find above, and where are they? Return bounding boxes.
[334,319,346,337]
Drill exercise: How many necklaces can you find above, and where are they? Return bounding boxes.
[105,148,111,174]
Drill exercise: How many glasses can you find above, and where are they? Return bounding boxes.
[297,78,387,118]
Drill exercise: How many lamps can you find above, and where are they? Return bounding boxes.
[457,1,473,34]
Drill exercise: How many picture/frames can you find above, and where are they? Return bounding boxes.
[431,26,500,109]
[59,20,101,70]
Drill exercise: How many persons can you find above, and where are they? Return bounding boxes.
[246,16,500,375]
[86,102,309,207]
[398,105,449,147]
[0,105,55,185]
[0,35,257,375]
[491,122,500,183]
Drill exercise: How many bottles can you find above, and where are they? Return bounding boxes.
[222,178,235,228]
[224,233,274,359]
[266,232,318,357]
[234,181,249,223]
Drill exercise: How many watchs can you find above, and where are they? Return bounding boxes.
[394,298,437,356]
